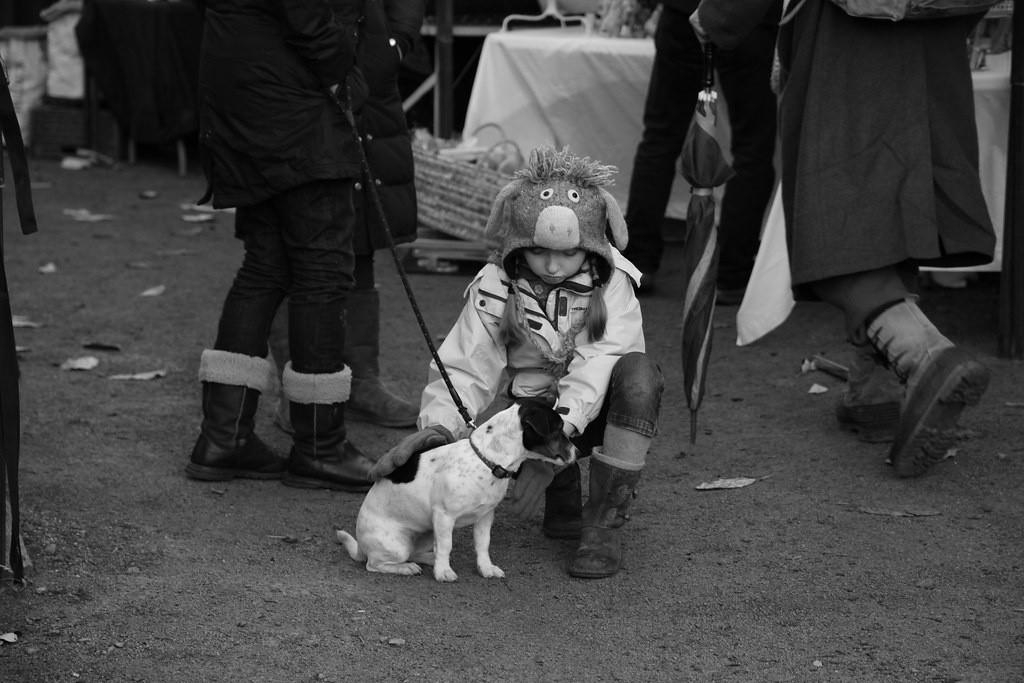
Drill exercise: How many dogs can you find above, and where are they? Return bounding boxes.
[336,397,580,583]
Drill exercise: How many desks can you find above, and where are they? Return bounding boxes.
[462,26,1012,288]
[419,23,541,139]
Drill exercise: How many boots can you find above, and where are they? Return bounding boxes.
[185,349,285,480]
[866,299,990,476]
[281,360,382,492]
[270,300,298,436]
[571,445,648,579]
[542,464,587,538]
[837,342,904,441]
[343,288,421,427]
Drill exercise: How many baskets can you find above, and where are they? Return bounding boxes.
[413,124,523,242]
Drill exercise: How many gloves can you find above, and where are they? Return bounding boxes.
[512,438,576,522]
[367,425,455,483]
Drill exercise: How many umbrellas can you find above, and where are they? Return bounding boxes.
[680,39,740,442]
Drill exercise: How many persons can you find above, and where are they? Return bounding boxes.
[186,0,386,494]
[616,0,785,306]
[0,0,222,182]
[245,2,436,447]
[369,172,665,578]
[689,0,998,479]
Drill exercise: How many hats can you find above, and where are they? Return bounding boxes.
[483,139,629,400]
